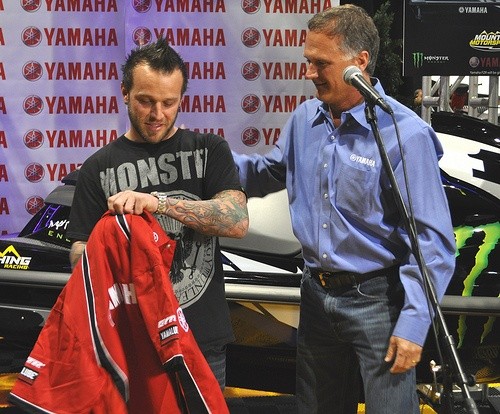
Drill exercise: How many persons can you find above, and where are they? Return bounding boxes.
[66,35,249,394]
[225,4,456,414]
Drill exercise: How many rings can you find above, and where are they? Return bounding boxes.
[411,359,418,364]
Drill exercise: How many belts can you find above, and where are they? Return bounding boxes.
[310,267,360,290]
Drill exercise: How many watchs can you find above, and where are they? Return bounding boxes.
[151,191,167,216]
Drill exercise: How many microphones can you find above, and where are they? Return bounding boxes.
[343,65,393,114]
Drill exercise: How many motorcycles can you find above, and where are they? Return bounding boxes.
[0,112,500,374]
